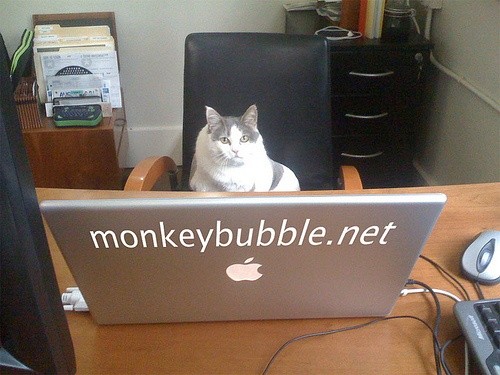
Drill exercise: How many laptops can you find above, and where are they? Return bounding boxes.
[39,192,447,328]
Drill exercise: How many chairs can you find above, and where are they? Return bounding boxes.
[121,33,362,192]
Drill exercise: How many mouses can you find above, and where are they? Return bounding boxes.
[459,230,500,284]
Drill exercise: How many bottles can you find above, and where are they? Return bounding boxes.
[381,7,416,43]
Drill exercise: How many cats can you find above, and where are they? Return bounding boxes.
[187,104,301,192]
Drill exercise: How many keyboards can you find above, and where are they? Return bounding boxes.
[454,298,500,375]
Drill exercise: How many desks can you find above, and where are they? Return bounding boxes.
[33,183,500,375]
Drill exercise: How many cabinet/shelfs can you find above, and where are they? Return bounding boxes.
[14,76,128,190]
[285,32,434,173]
